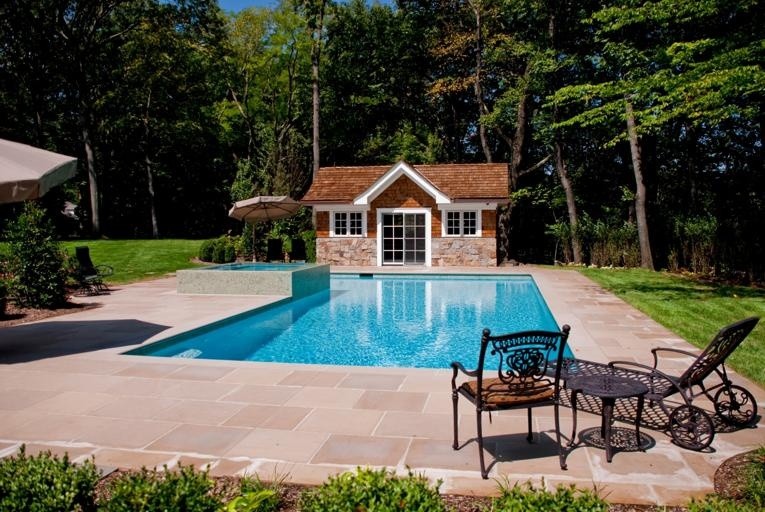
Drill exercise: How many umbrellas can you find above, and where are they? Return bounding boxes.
[0,138,78,202]
[228,194,302,258]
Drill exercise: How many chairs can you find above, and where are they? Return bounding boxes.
[288,238,308,262]
[267,238,285,263]
[75,246,114,296]
